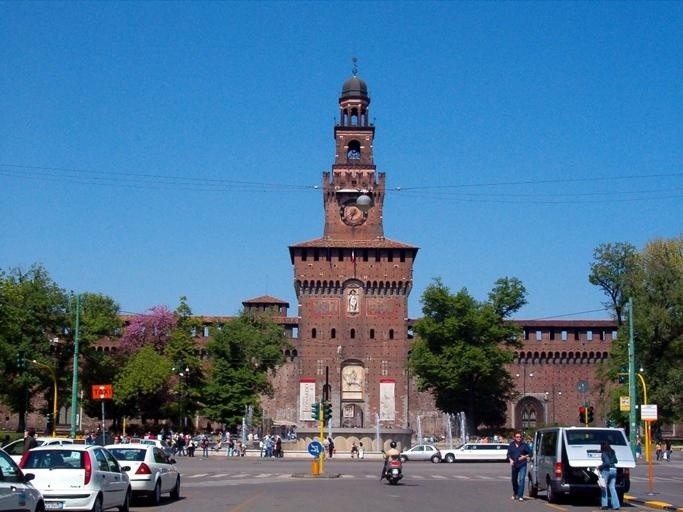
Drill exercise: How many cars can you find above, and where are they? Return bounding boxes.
[440,443,511,463]
[18,445,132,512]
[400,444,441,464]
[126,438,172,454]
[105,443,181,503]
[0,447,45,512]
[2,437,74,467]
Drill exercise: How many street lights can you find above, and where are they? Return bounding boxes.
[514,363,534,431]
[178,366,190,431]
[546,391,563,424]
[578,383,586,405]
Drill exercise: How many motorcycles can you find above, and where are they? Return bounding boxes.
[379,450,404,484]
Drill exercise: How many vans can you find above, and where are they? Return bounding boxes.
[526,426,637,504]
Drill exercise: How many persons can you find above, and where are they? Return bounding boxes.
[82,427,285,458]
[595,439,621,509]
[507,431,532,500]
[525,434,533,462]
[327,437,334,457]
[358,442,364,459]
[347,289,358,316]
[655,432,673,462]
[22,427,38,455]
[343,369,362,388]
[350,441,358,458]
[422,433,510,443]
[635,438,642,459]
[378,441,402,481]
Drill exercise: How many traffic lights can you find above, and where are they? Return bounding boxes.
[323,403,333,421]
[587,406,594,423]
[15,349,25,369]
[579,406,586,424]
[310,402,319,420]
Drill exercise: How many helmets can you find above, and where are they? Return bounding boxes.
[390,442,396,447]
